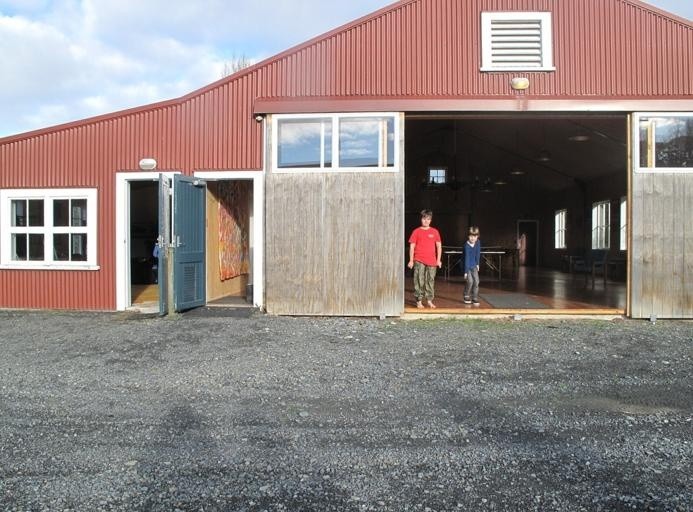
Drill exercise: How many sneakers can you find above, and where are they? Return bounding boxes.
[463,297,480,305]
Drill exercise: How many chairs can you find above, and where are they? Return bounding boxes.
[575,249,607,288]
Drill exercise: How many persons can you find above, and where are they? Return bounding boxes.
[461,225,481,307]
[406,209,443,309]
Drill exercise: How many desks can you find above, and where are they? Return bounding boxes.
[443,250,505,280]
